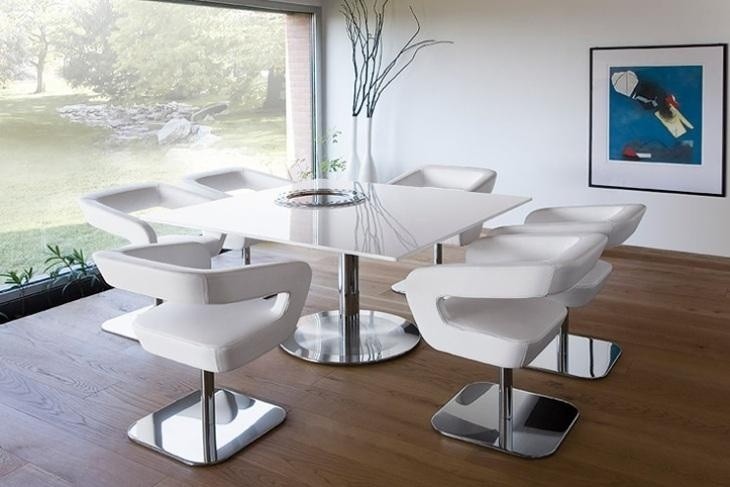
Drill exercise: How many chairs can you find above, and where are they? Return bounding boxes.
[176,164,302,303]
[483,199,646,383]
[82,179,231,347]
[87,238,314,466]
[385,230,611,464]
[379,165,500,343]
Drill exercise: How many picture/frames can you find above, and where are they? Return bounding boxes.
[589,43,727,199]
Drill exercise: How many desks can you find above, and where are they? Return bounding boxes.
[137,175,536,370]
